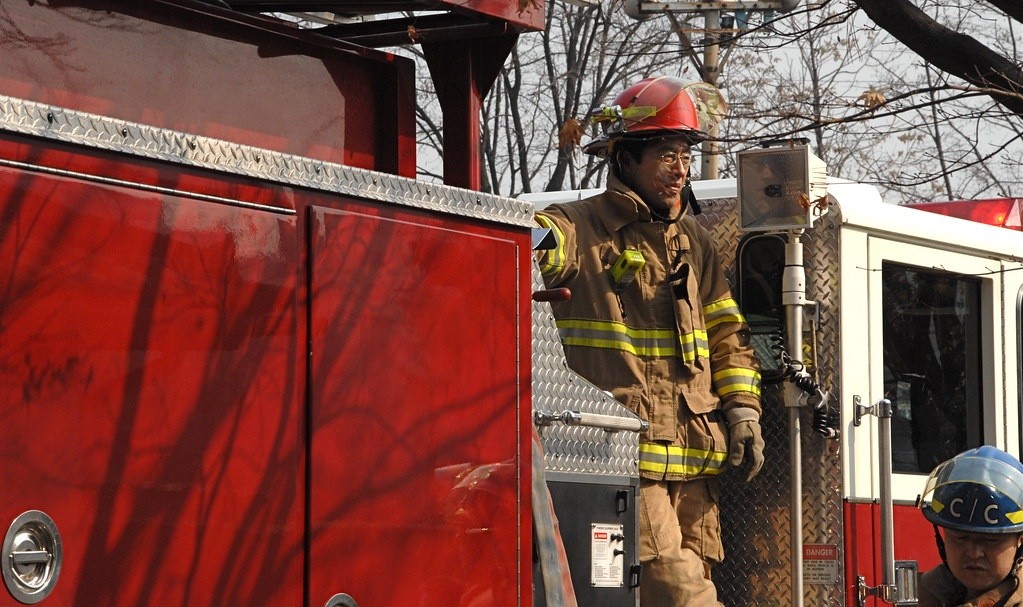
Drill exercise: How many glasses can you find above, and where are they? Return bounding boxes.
[639,150,696,167]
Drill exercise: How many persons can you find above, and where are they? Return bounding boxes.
[914,444,1023,607]
[530,78,765,606]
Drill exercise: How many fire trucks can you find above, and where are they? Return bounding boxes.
[0,0,1022,607]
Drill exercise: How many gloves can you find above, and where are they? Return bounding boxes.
[726,406,765,485]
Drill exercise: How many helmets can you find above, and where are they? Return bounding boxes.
[915,445,1023,534]
[591,77,712,140]
